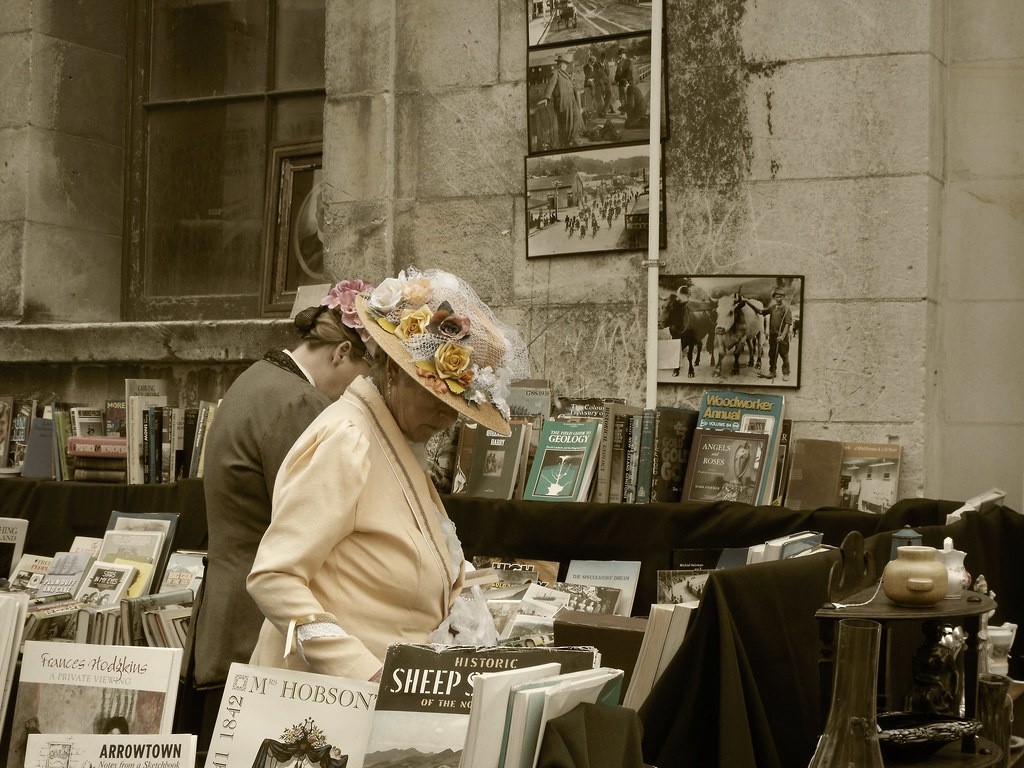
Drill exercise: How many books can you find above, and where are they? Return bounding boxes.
[422,379,1007,525]
[0,379,224,768]
[202,530,841,768]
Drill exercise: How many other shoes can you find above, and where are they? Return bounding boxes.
[764,371,777,379]
[782,371,789,381]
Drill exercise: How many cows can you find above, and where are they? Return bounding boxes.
[658,285,765,378]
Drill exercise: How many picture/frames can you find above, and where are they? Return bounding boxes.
[653,275,805,391]
[523,139,668,261]
[525,0,667,48]
[526,31,670,155]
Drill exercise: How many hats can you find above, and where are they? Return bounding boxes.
[771,289,785,299]
[356,265,532,436]
[321,279,387,364]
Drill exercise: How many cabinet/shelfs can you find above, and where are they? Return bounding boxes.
[800,573,1004,768]
[0,476,995,768]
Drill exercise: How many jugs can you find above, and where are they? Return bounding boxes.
[889,524,922,560]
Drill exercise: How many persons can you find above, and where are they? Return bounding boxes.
[566,0,573,5]
[537,47,650,147]
[743,290,799,381]
[248,263,533,682]
[531,183,644,239]
[179,279,376,768]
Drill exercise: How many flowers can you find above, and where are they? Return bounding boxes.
[319,267,513,423]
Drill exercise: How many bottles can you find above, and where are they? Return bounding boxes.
[882,545,949,607]
[977,673,1014,768]
[811,618,884,768]
